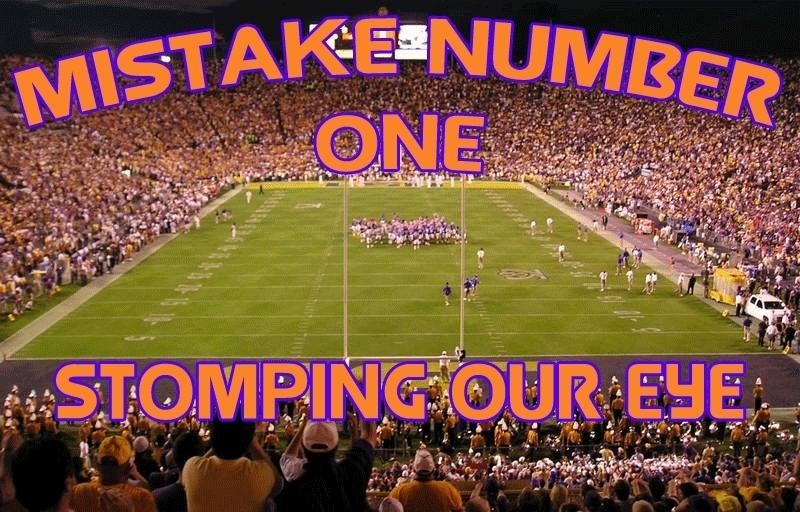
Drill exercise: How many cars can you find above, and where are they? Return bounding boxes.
[739,291,792,329]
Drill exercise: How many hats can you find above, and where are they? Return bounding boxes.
[2,381,799,508]
